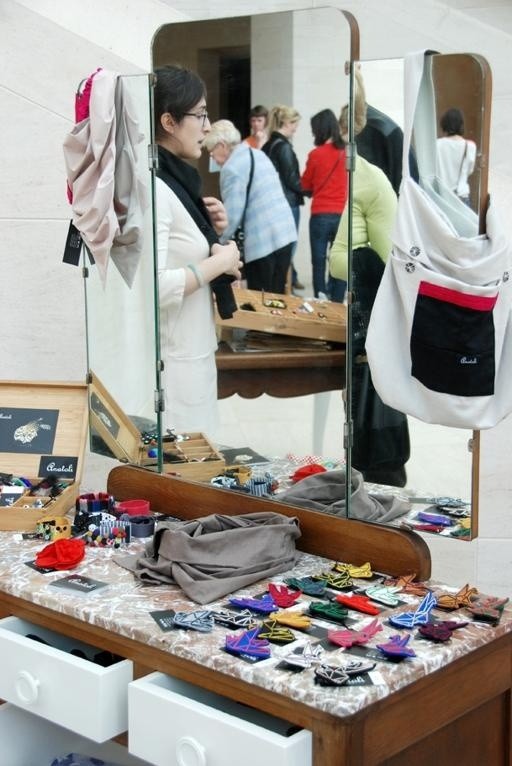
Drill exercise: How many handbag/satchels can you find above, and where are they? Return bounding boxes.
[414,56,478,238]
[365,50,510,430]
[231,148,255,265]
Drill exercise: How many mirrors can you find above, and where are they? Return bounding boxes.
[75,6,494,543]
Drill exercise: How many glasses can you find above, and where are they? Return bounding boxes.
[169,109,208,125]
[208,144,219,155]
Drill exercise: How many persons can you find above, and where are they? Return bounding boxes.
[304,112,355,299]
[266,107,304,225]
[246,107,267,144]
[437,111,479,197]
[340,63,409,488]
[145,65,243,438]
[202,120,299,291]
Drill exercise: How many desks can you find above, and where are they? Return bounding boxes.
[0,528,512,764]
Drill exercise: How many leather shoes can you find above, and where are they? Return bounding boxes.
[293,281,303,288]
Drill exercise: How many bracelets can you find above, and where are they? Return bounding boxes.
[185,261,203,288]
